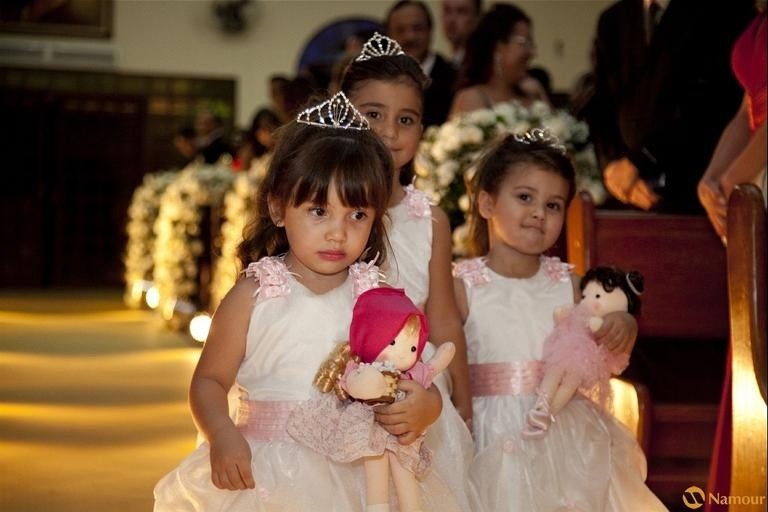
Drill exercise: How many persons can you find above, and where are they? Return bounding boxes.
[154,92,479,512]
[336,31,474,436]
[451,127,673,512]
[697,6,766,512]
[590,0,761,404]
[171,0,593,228]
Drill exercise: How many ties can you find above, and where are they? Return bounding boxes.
[646,1,663,46]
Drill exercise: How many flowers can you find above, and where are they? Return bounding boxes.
[413,99,607,256]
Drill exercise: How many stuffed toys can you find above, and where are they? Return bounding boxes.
[521,268,644,439]
[287,286,456,512]
